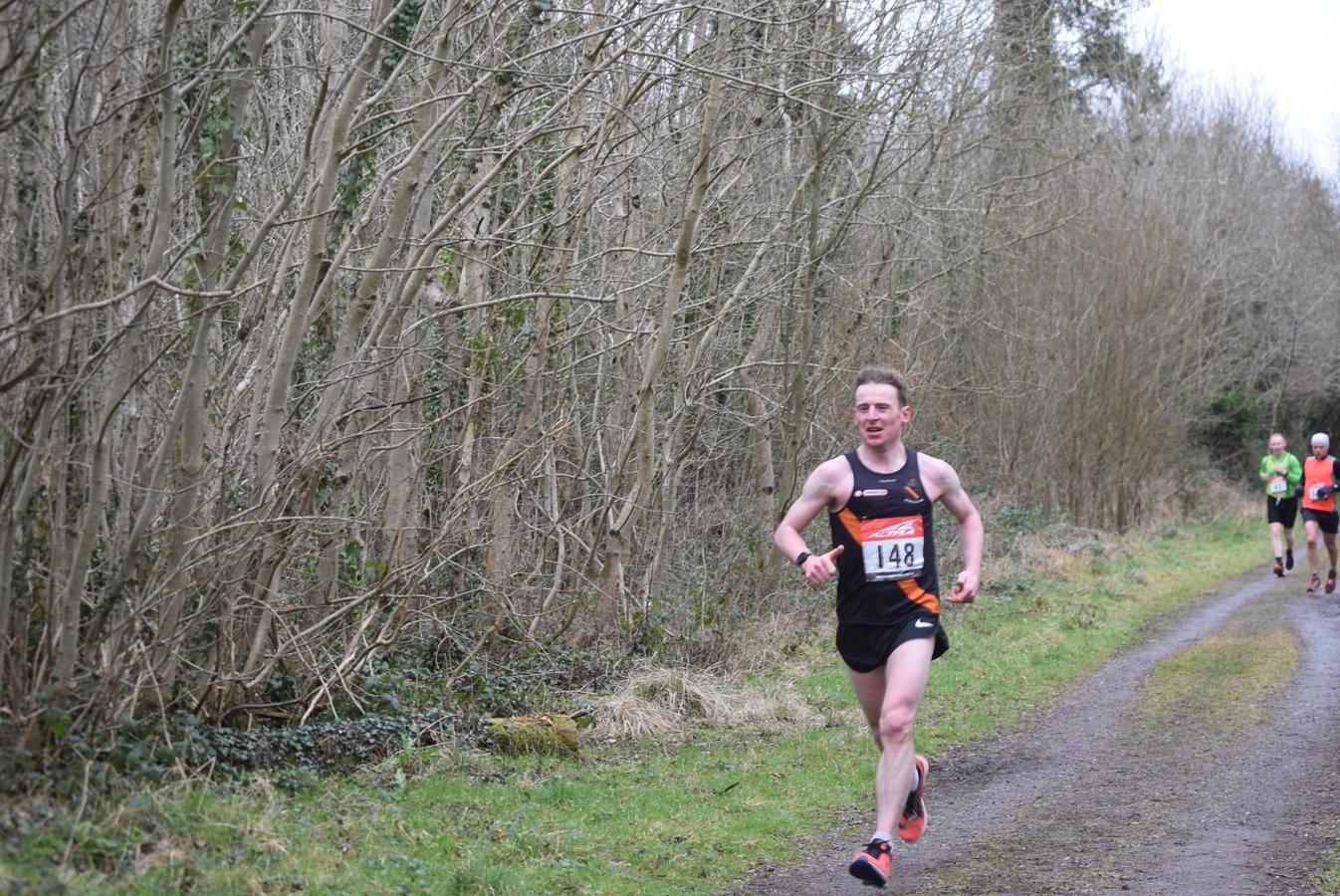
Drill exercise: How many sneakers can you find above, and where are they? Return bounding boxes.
[896,756,929,842]
[1274,561,1284,577]
[849,839,893,886]
[1286,550,1293,569]
[1308,575,1321,591]
[1325,571,1337,593]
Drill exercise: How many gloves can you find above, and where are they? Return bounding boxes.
[1296,485,1304,498]
[1317,487,1333,499]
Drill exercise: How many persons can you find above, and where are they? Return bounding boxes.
[775,369,985,887]
[1257,433,1303,576]
[1295,433,1340,595]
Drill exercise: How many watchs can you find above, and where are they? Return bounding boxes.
[795,551,814,567]
